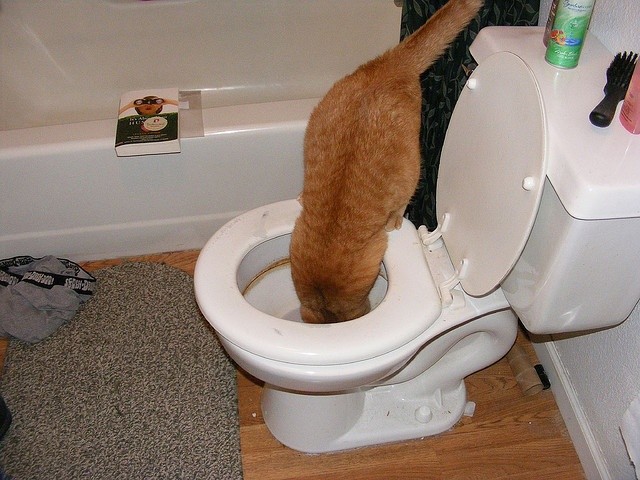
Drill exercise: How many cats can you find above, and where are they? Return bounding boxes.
[286,0,484,325]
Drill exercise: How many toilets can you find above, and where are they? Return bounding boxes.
[194,22,640,454]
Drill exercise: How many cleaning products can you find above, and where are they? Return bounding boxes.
[544,0,597,70]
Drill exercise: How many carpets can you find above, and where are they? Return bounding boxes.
[2,259,243,479]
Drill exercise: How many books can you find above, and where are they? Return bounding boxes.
[115,87,180,157]
